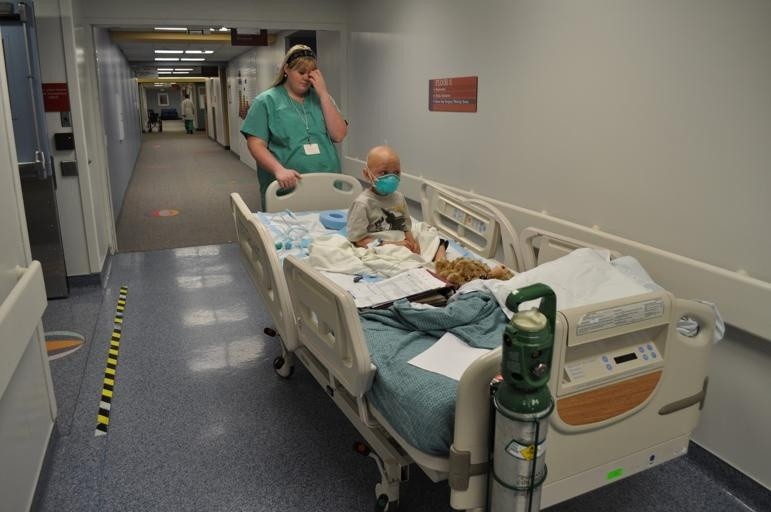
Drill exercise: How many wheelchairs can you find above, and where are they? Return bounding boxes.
[147,109,162,133]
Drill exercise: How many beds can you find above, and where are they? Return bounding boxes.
[229,171,726,512]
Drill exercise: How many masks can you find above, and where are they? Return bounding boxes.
[366,165,400,196]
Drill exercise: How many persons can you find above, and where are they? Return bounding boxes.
[347,146,449,265]
[180,94,194,134]
[239,44,349,214]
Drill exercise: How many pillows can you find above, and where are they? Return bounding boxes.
[493,247,654,314]
